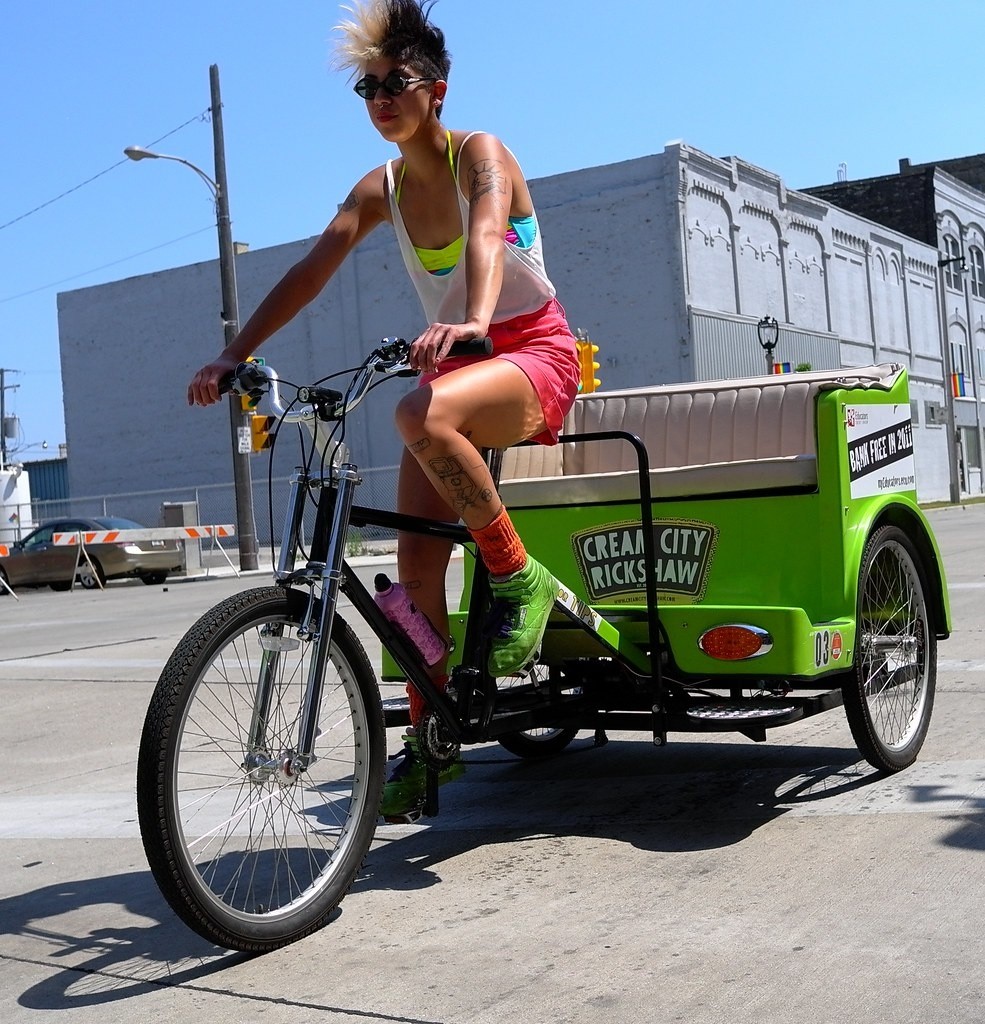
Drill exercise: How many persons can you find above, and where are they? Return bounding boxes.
[187,0,580,816]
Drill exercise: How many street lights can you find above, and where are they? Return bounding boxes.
[758,315,780,374]
[124,146,259,571]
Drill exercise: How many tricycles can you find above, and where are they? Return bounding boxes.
[136,337,952,957]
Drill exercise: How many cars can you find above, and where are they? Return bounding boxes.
[0,515,185,595]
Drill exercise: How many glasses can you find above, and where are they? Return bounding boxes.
[353,74,436,100]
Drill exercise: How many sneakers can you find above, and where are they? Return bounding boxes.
[488,553,559,676]
[379,727,467,815]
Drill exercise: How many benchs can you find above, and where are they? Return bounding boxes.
[489,363,905,510]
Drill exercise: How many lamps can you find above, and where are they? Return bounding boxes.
[939,255,969,280]
